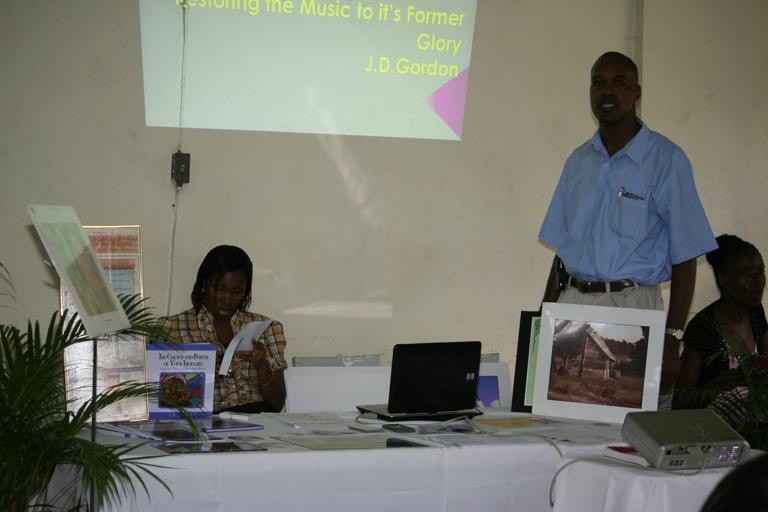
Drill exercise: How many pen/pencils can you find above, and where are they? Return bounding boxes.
[618,186,624,198]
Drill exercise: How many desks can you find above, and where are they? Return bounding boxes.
[28,409,628,512]
[551,448,768,512]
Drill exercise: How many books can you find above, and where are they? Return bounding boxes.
[604,445,652,467]
[473,415,586,432]
[219,319,272,378]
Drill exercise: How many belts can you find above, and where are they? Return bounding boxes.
[570,277,634,292]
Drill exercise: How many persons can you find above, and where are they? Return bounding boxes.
[151,244,288,413]
[672,232,768,450]
[539,52,719,409]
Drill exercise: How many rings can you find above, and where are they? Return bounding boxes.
[250,350,256,358]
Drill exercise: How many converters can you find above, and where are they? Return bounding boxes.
[172,153,190,187]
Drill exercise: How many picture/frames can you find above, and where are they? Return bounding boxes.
[474,361,514,411]
[531,303,665,424]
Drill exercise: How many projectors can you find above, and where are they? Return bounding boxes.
[621,409,750,470]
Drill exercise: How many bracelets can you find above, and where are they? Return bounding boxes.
[259,376,273,386]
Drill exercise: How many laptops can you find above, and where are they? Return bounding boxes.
[356,341,484,421]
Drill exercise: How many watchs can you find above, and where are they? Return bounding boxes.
[666,326,685,341]
